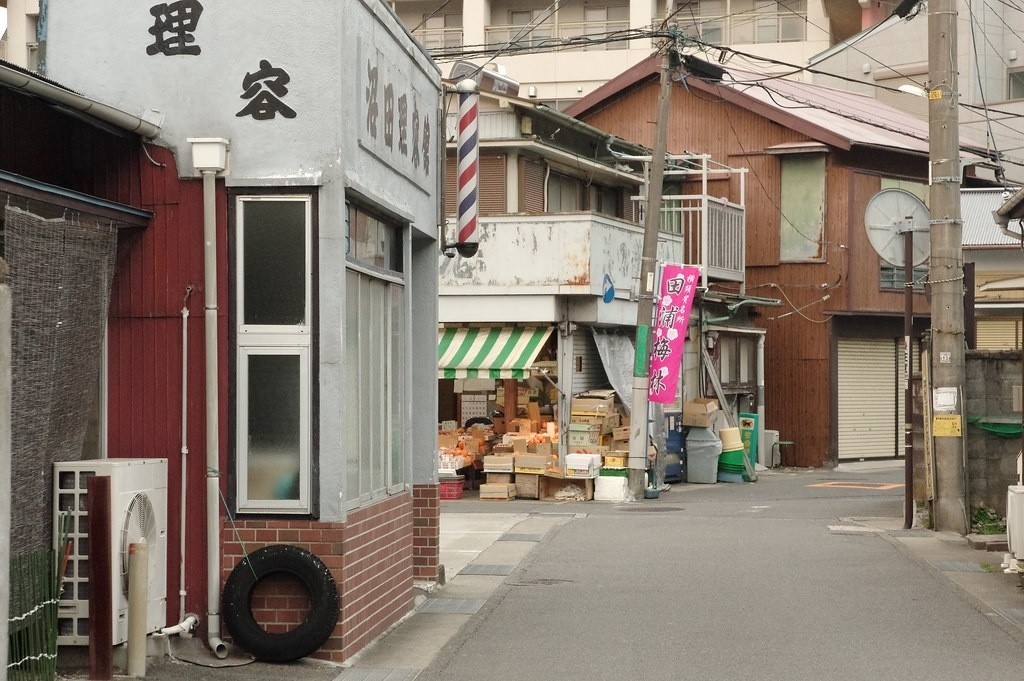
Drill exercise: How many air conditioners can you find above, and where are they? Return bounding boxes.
[50,456,169,647]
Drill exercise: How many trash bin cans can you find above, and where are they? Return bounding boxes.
[685,427,723,483]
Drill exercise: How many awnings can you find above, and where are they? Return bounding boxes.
[438,326,554,382]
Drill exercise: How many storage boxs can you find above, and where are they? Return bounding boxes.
[439,389,630,501]
[681,398,720,427]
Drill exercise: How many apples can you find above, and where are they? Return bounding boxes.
[528,434,545,444]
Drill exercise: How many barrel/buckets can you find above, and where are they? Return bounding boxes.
[718,427,746,482]
